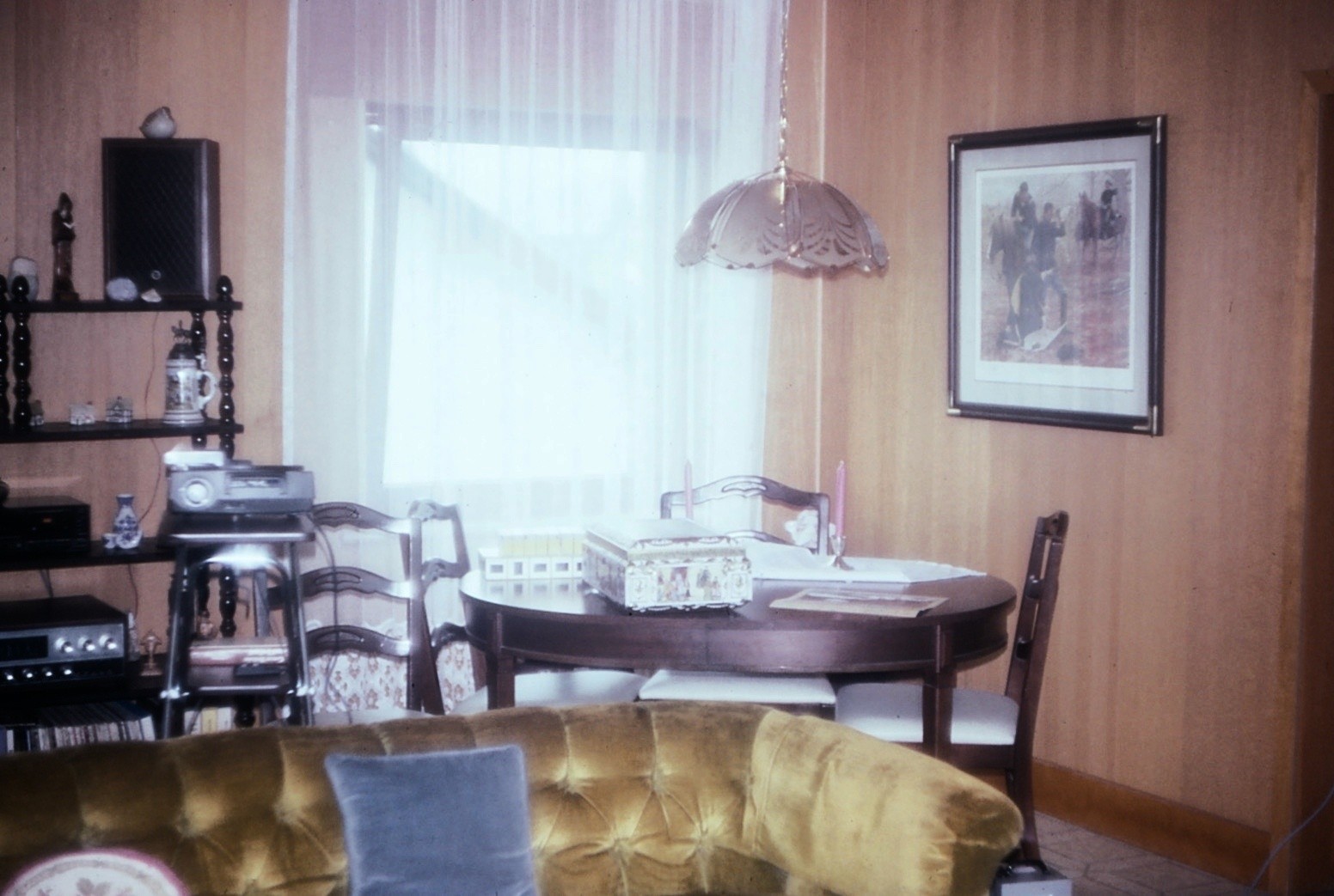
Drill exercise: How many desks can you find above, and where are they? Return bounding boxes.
[456,552,1018,766]
[161,511,316,738]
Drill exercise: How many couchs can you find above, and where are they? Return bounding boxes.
[0,697,1027,896]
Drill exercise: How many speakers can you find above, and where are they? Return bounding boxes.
[98,136,221,301]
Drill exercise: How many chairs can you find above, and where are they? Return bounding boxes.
[637,473,838,722]
[268,501,438,726]
[836,509,1069,867]
[397,498,650,717]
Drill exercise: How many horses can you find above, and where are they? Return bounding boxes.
[987,213,1026,299]
[1075,191,1128,266]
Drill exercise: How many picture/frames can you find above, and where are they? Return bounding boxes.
[947,112,1165,438]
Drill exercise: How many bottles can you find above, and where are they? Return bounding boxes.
[113,493,142,549]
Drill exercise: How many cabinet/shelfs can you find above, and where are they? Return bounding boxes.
[0,274,255,727]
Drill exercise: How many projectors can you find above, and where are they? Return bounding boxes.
[163,464,317,518]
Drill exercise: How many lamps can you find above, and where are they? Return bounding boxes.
[671,0,890,283]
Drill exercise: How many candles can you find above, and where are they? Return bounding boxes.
[836,458,846,537]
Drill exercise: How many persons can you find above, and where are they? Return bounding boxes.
[1010,184,1048,339]
[1100,178,1120,228]
[1028,202,1075,333]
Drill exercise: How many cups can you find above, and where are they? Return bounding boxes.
[161,342,216,426]
[101,533,116,548]
[70,399,95,425]
[106,396,133,424]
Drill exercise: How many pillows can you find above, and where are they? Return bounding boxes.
[323,744,540,896]
